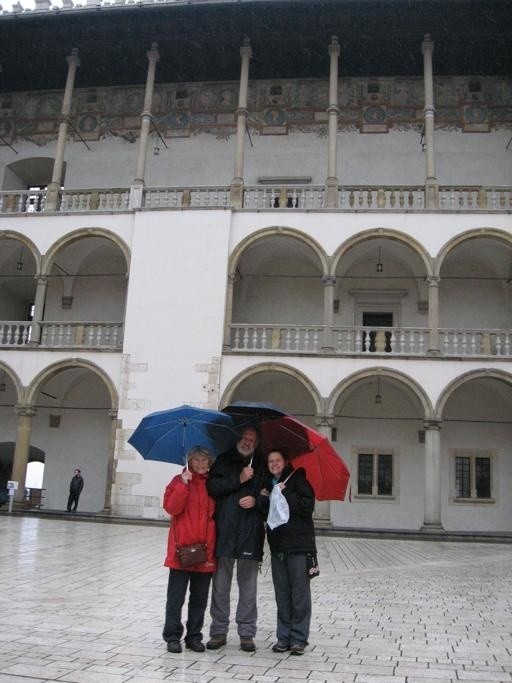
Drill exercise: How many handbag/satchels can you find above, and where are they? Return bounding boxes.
[176,541,209,568]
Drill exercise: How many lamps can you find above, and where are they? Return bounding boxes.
[152,131,162,155]
[374,375,381,404]
[376,244,383,272]
[16,245,26,271]
[421,131,427,151]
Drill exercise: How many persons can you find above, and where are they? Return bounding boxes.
[206,429,273,652]
[64,469,84,512]
[162,446,217,653]
[260,450,316,657]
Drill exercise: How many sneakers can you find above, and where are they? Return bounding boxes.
[290,643,307,656]
[167,641,182,653]
[206,634,226,649]
[186,638,205,652]
[271,639,291,652]
[240,636,255,651]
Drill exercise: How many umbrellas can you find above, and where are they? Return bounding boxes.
[127,406,232,471]
[205,400,287,469]
[259,415,351,502]
